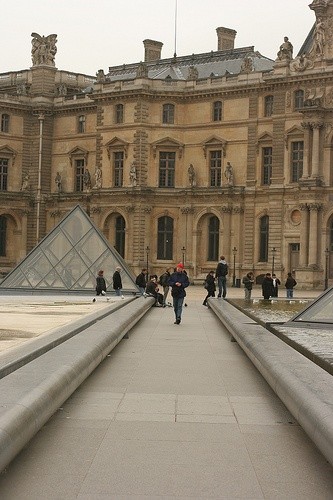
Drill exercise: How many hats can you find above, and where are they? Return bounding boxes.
[177,264,184,269]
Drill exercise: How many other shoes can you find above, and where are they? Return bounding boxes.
[203,303,207,306]
[177,317,181,323]
[174,321,177,324]
[218,295,221,297]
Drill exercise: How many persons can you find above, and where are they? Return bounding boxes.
[137,263,190,325]
[285,272,297,297]
[37,41,48,64]
[243,271,255,299]
[261,272,280,299]
[203,256,229,306]
[224,162,233,185]
[113,267,125,299]
[187,164,195,186]
[276,37,293,61]
[92,270,111,302]
[55,172,62,192]
[83,169,91,190]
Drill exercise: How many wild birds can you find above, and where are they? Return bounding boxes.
[121,295,125,299]
[185,303,188,307]
[106,298,111,301]
[93,298,96,302]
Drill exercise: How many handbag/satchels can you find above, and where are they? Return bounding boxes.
[102,290,106,295]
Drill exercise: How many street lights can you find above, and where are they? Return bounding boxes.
[271,247,277,276]
[323,248,329,290]
[145,245,151,284]
[181,246,186,265]
[232,246,237,286]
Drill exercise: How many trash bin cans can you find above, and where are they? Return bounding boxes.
[236,279,241,288]
[256,274,265,284]
